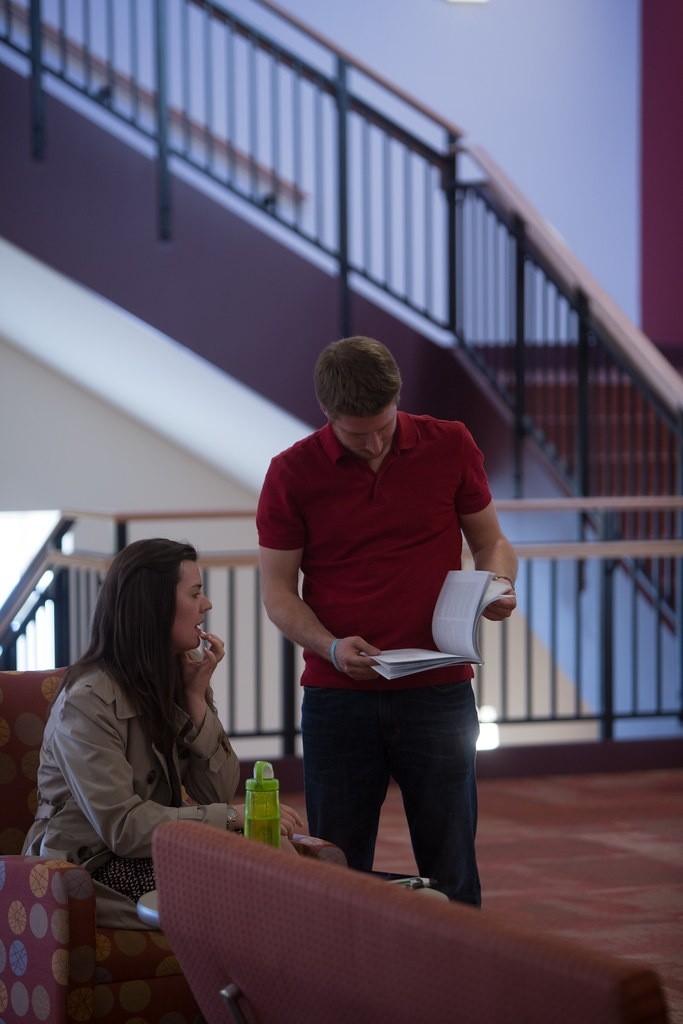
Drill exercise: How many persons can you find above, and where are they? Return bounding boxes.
[21,537,304,929]
[257,336,519,909]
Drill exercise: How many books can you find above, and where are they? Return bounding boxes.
[359,570,516,681]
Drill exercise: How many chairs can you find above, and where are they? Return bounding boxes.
[151,822,672,1024]
[0,668,346,1024]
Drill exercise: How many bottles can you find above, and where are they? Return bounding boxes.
[243,761,282,849]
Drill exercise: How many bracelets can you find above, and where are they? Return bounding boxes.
[493,576,515,591]
[330,638,342,674]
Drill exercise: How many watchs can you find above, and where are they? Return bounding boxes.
[226,805,237,832]
